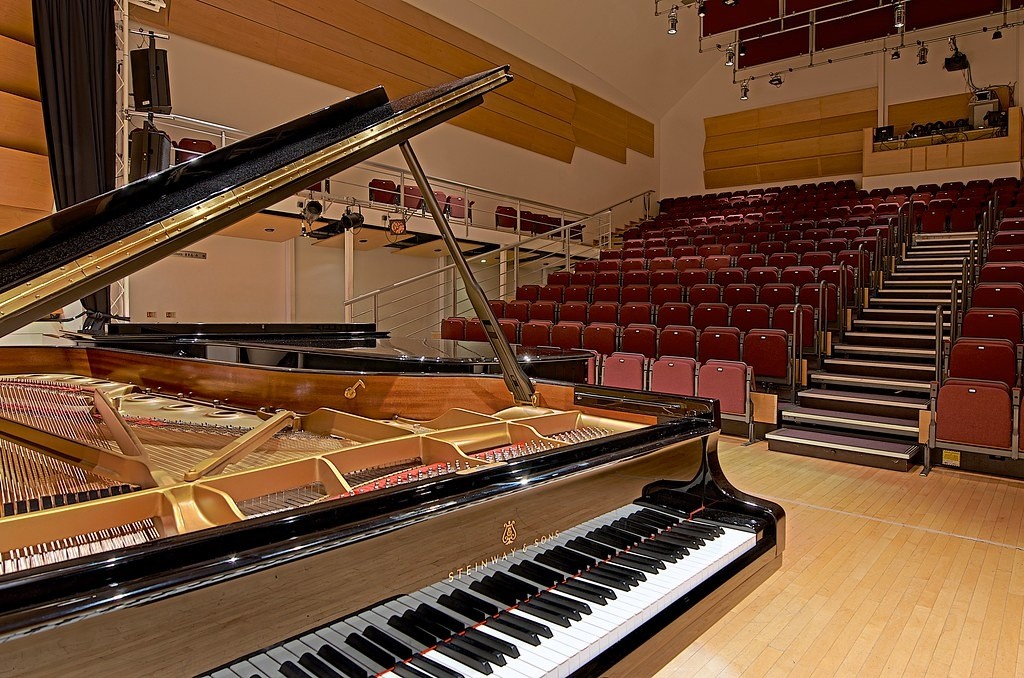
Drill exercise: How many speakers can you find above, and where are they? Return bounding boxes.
[944,54,968,72]
[130,128,171,181]
[130,48,173,116]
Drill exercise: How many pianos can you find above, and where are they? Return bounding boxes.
[57,322,596,385]
[0,64,789,678]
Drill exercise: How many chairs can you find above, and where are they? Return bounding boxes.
[913,119,970,138]
[172,137,217,167]
[368,179,475,225]
[441,177,1024,474]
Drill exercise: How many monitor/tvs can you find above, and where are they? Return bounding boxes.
[875,125,894,142]
[988,112,1008,127]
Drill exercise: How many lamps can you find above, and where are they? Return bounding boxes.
[916,40,928,65]
[769,73,783,85]
[740,76,754,101]
[893,0,907,28]
[740,43,747,57]
[891,50,900,60]
[716,43,735,66]
[667,4,679,35]
[698,0,706,17]
[992,30,1002,40]
